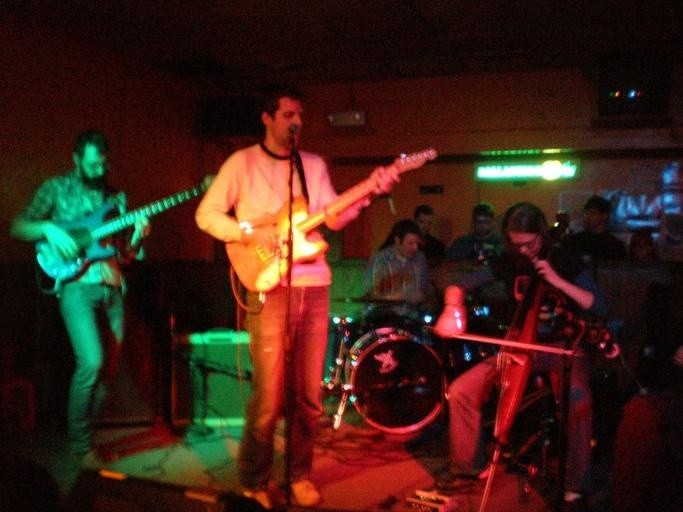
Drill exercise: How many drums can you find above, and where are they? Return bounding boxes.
[345,327,447,435]
[446,308,503,382]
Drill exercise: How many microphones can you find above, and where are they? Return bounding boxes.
[289,123,296,150]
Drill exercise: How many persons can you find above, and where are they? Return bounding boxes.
[365,195,682,315]
[7,128,153,472]
[429,200,608,512]
[194,83,400,510]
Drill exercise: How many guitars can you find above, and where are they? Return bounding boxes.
[36,176,215,281]
[226,149,438,294]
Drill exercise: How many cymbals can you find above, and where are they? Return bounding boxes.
[351,294,406,304]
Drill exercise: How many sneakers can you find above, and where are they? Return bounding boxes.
[243,490,272,509]
[413,477,472,500]
[290,480,320,507]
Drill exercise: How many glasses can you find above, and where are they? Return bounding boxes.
[508,235,538,252]
